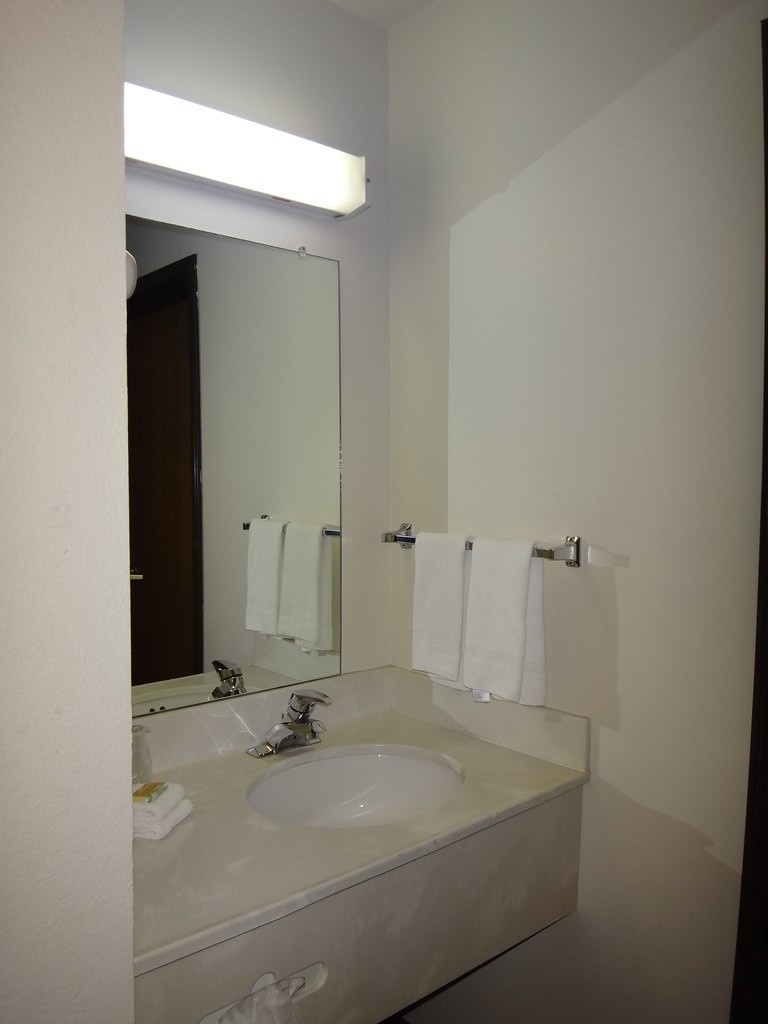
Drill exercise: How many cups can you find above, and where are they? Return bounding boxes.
[131,726,150,784]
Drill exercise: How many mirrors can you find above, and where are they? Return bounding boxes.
[125,214,342,718]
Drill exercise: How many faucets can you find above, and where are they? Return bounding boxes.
[209,660,246,699]
[246,687,333,758]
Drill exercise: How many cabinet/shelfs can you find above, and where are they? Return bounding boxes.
[134,784,585,1024]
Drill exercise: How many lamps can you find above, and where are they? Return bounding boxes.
[124,80,372,220]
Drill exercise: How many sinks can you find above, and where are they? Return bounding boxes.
[133,685,266,718]
[246,742,467,831]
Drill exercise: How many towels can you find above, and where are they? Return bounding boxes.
[133,781,193,841]
[461,534,546,706]
[244,519,287,640]
[412,532,471,692]
[278,522,333,653]
